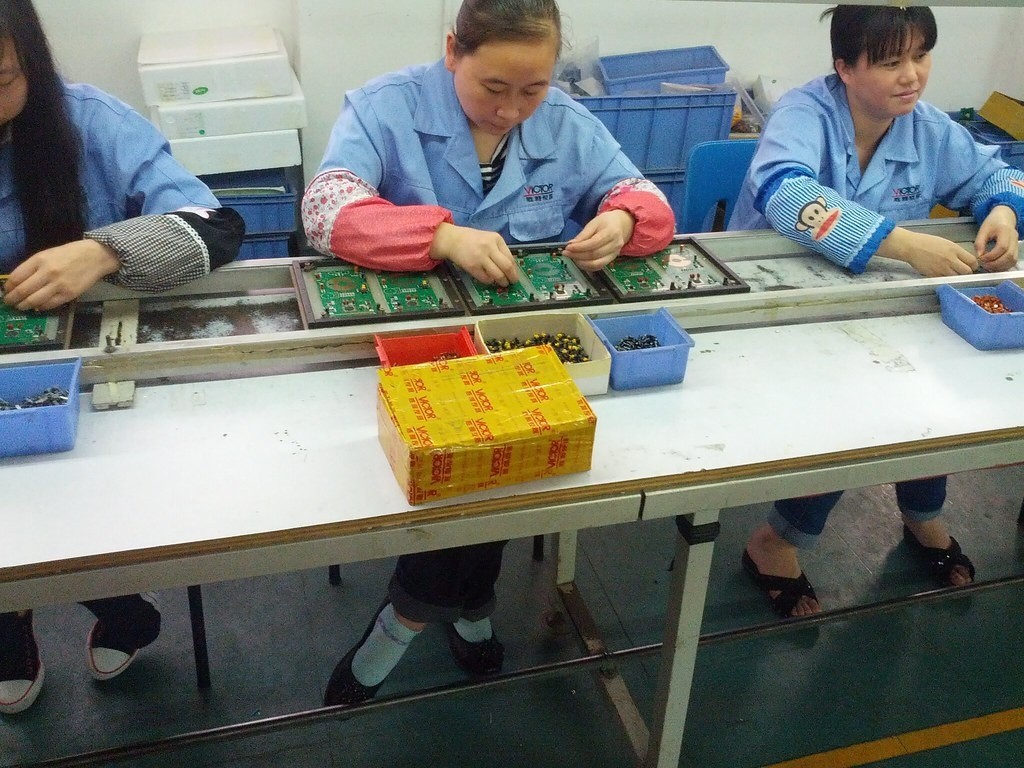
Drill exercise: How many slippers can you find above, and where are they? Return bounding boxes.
[903,520,975,586]
[742,547,822,618]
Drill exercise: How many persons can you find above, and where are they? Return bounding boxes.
[719,4,1024,619]
[300,1,674,721]
[1,0,244,715]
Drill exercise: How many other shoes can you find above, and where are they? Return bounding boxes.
[324,597,398,721]
[442,622,505,676]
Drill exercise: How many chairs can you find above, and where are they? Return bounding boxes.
[680,138,761,233]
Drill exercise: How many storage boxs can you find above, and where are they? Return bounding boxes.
[572,86,738,172]
[945,92,1024,173]
[150,107,302,176]
[376,345,598,505]
[232,232,293,262]
[473,312,612,397]
[374,324,479,367]
[936,279,1023,352]
[584,306,695,390]
[197,171,299,236]
[136,22,294,107]
[562,172,687,243]
[1,357,84,460]
[596,45,729,97]
[156,68,308,140]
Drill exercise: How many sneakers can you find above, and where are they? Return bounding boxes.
[85,618,142,681]
[0,610,45,714]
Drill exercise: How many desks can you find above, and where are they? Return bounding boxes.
[0,215,1023,768]
